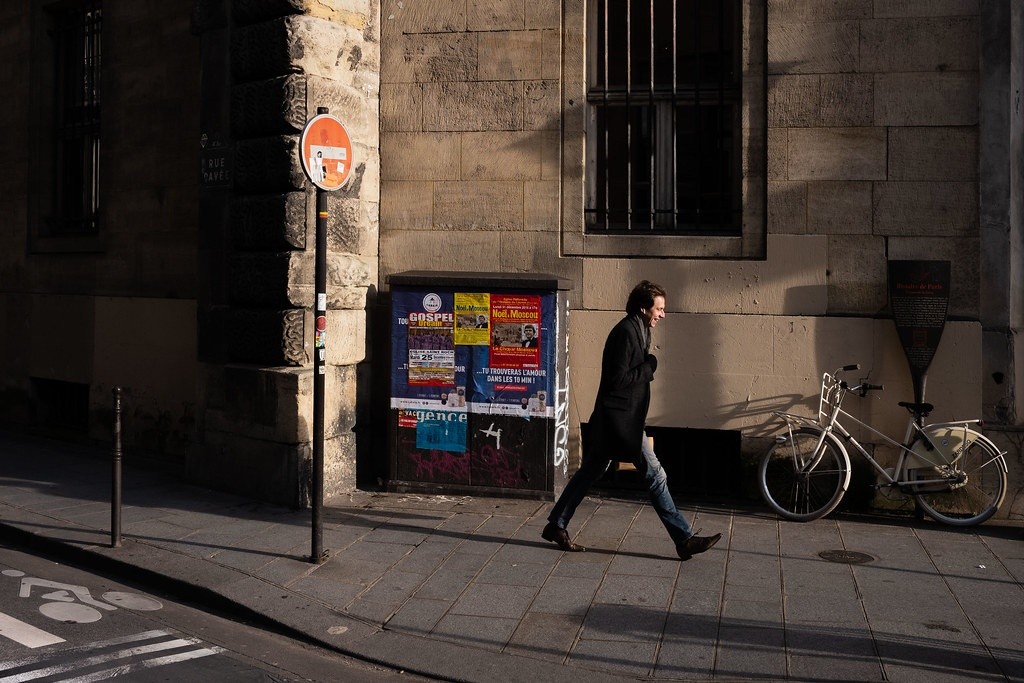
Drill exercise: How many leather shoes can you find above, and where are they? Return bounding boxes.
[540,520,585,550]
[675,532,722,560]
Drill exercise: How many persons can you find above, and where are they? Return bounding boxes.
[541,278,721,561]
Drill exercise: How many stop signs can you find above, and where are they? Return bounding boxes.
[299,113,355,191]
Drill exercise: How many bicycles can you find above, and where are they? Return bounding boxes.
[757,364,1009,524]
